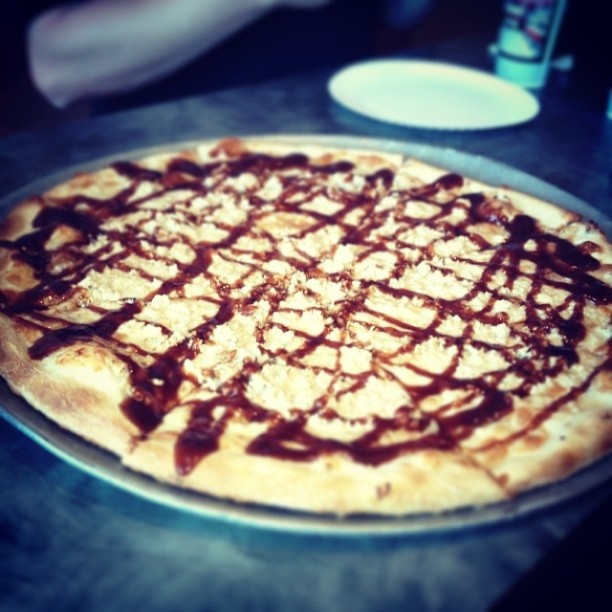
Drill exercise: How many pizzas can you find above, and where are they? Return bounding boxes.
[0,139,612,515]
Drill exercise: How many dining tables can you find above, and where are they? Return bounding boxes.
[2,43,610,612]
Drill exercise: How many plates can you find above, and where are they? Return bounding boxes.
[328,57,541,132]
[0,136,612,540]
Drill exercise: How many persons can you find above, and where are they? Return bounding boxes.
[28,1,437,108]
